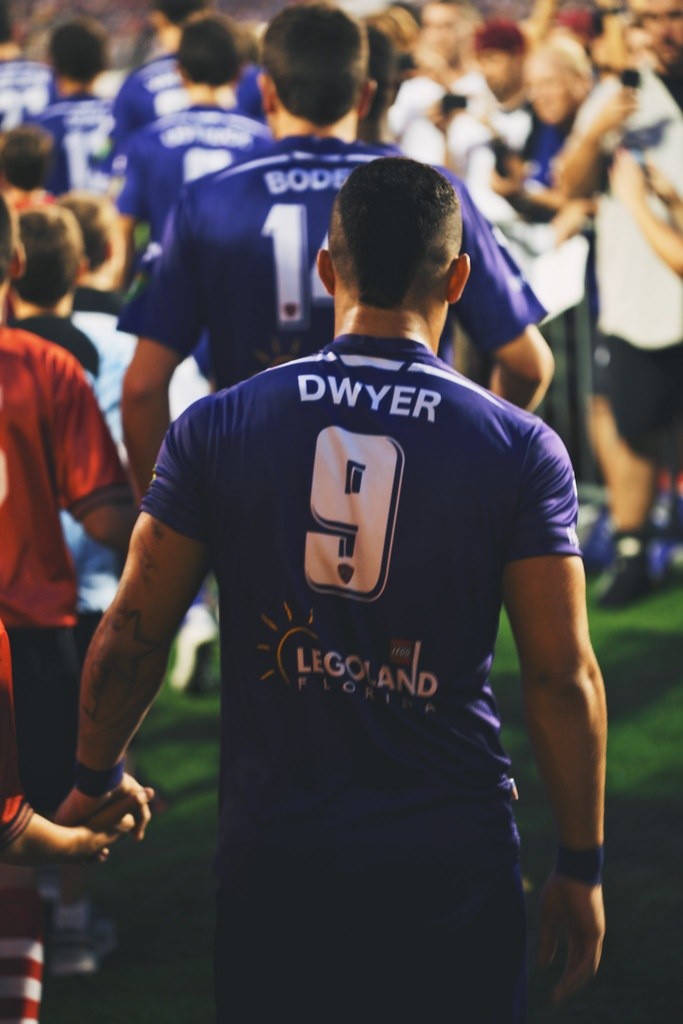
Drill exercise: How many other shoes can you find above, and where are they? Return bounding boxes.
[599,528,655,608]
[48,916,114,975]
[166,611,218,695]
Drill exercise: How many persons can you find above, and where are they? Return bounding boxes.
[0,0,683,1024]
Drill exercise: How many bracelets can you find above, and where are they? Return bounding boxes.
[74,760,123,797]
[557,845,606,886]
